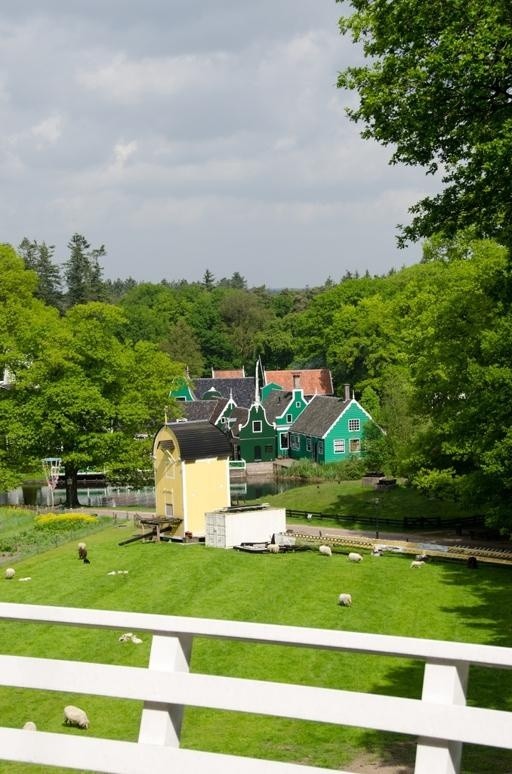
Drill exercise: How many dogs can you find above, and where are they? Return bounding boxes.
[409,561,426,569]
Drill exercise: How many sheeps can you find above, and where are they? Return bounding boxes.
[22,720,37,733]
[318,544,332,557]
[62,705,90,730]
[338,593,352,608]
[348,551,362,563]
[267,544,280,555]
